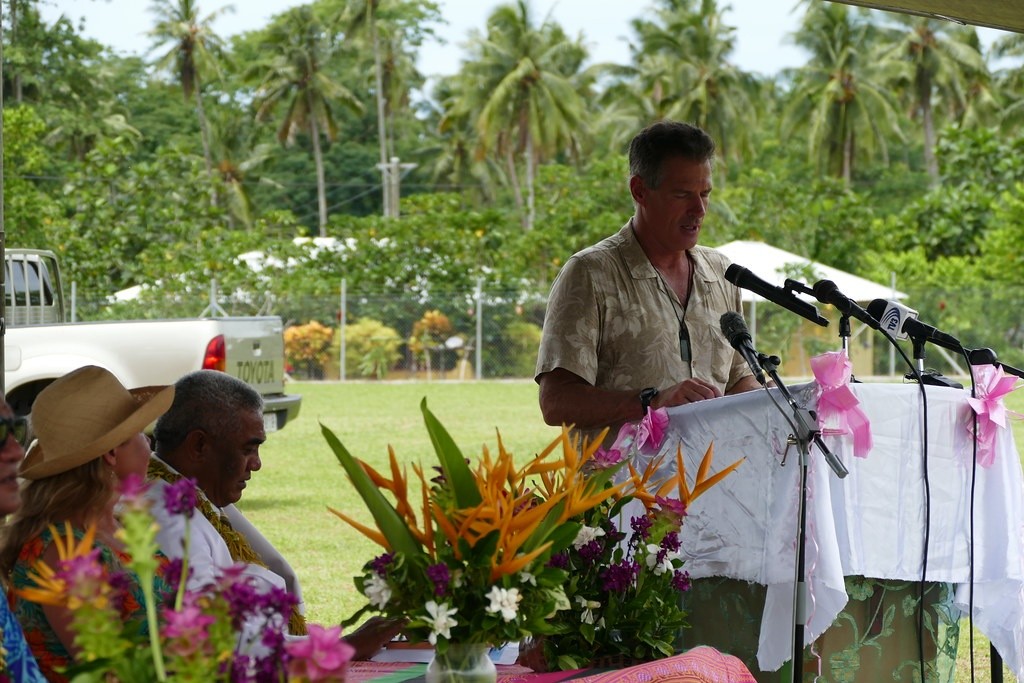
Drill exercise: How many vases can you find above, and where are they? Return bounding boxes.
[519,621,549,673]
[426,643,496,683]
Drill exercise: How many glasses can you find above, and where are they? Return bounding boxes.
[0,417,28,448]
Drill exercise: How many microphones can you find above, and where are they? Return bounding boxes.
[720,312,766,387]
[814,280,880,330]
[868,300,960,345]
[725,263,829,330]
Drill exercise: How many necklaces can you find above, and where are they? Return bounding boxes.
[647,256,690,361]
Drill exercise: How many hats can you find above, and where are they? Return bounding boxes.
[17,365,175,480]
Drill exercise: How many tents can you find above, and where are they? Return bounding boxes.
[714,240,909,383]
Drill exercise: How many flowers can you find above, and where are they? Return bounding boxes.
[10,477,356,683]
[311,397,749,671]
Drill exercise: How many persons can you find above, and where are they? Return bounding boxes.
[0,366,307,683]
[535,121,778,481]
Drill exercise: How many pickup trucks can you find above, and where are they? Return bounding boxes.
[3,248,302,445]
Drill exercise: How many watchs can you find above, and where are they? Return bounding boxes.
[639,386,658,417]
